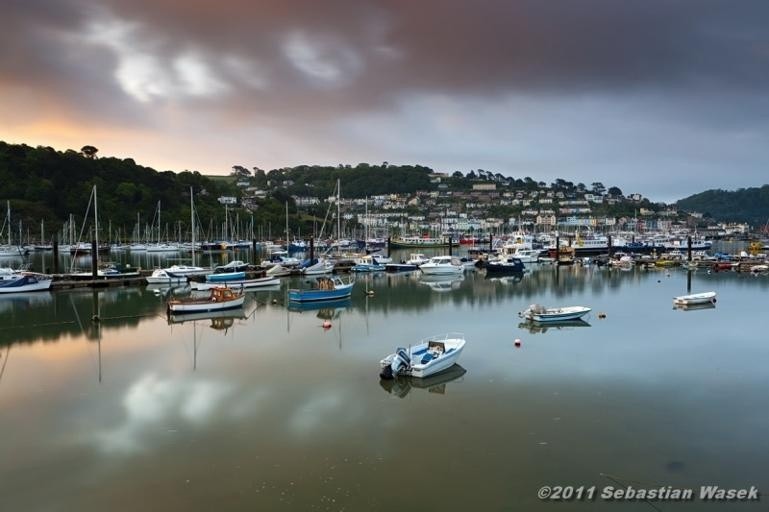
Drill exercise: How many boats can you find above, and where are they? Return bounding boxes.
[0,178,769,322]
[379,333,467,381]
